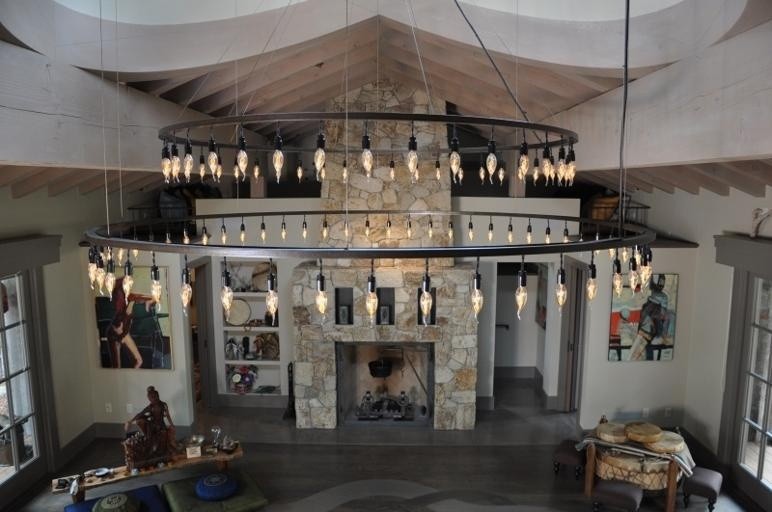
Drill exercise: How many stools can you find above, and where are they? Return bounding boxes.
[589,466,725,511]
[63,469,268,511]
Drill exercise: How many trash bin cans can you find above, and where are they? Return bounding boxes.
[0,413,28,467]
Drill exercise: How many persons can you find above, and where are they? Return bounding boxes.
[124,385,177,453]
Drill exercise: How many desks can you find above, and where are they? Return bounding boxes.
[51,440,244,501]
[583,425,691,511]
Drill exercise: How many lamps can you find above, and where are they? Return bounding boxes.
[86,0,654,325]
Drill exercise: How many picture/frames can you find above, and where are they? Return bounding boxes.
[93,267,173,371]
[609,273,679,363]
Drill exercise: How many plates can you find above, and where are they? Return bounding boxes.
[93,467,109,477]
